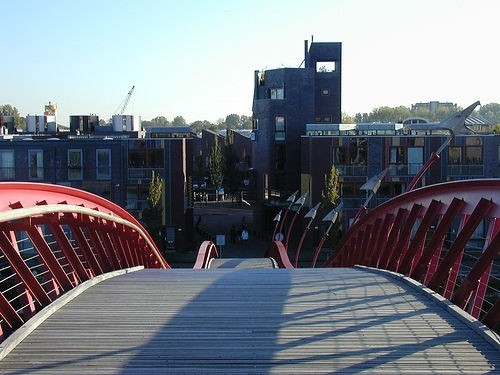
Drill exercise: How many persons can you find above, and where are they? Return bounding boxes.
[229,213,251,248]
